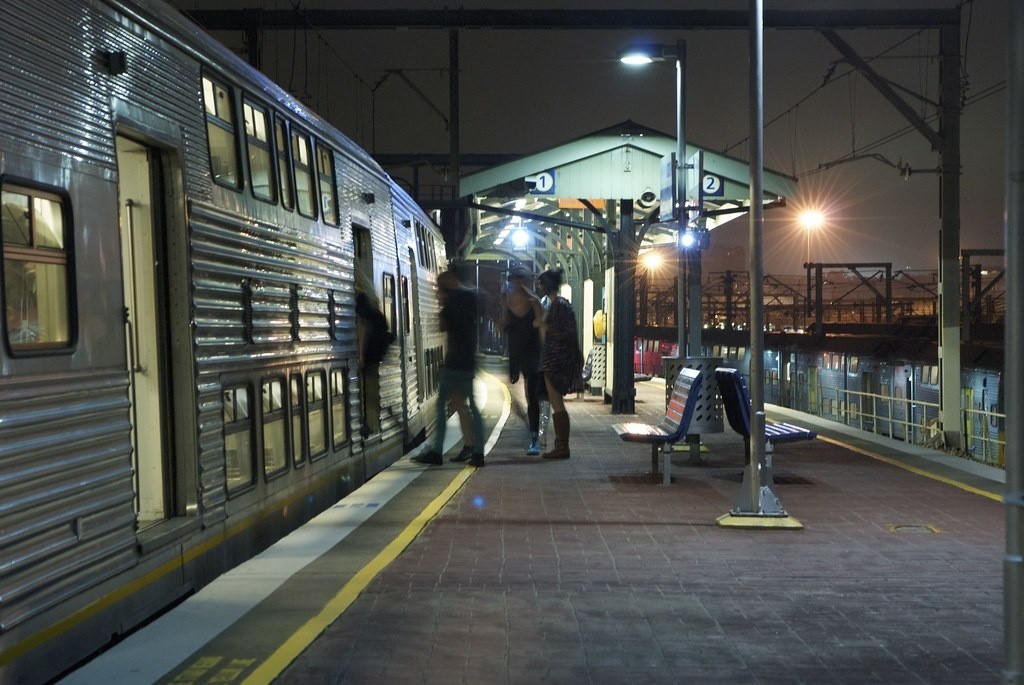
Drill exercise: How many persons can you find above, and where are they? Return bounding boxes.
[502,267,581,459]
[355,286,395,437]
[407,268,489,468]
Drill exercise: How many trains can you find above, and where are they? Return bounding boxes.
[0,0,449,685]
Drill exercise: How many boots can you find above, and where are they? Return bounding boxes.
[543,411,571,460]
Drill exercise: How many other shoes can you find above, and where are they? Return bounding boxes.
[449,445,472,460]
[410,445,444,465]
[527,438,540,454]
[469,453,485,466]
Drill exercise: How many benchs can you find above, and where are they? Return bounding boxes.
[609,367,703,488]
[576,353,592,401]
[714,368,819,486]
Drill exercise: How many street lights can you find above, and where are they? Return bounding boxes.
[617,39,689,446]
